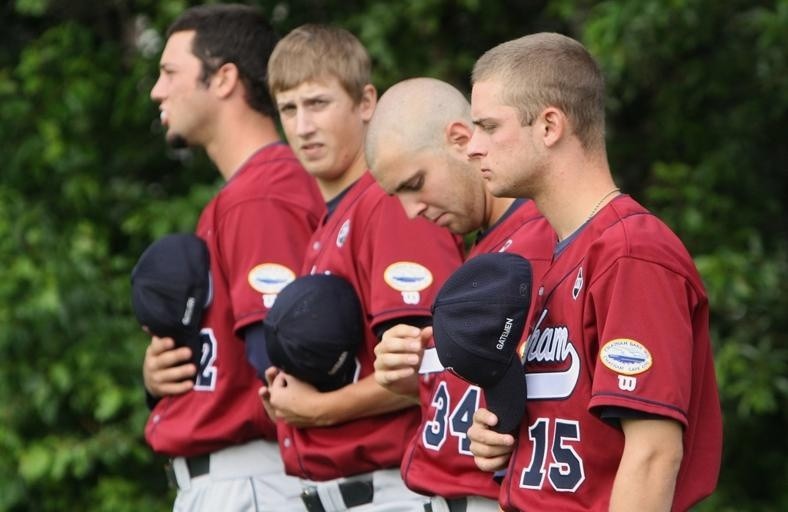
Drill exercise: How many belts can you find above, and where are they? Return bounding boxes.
[164,452,211,491]
[298,479,375,512]
[421,495,469,512]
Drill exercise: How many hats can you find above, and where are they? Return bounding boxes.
[427,251,534,437]
[260,272,367,394]
[128,230,212,391]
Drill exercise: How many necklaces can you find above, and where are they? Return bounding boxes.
[583,184,625,227]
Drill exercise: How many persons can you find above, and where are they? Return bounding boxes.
[258,19,467,512]
[366,75,563,512]
[465,24,726,512]
[140,2,327,512]
